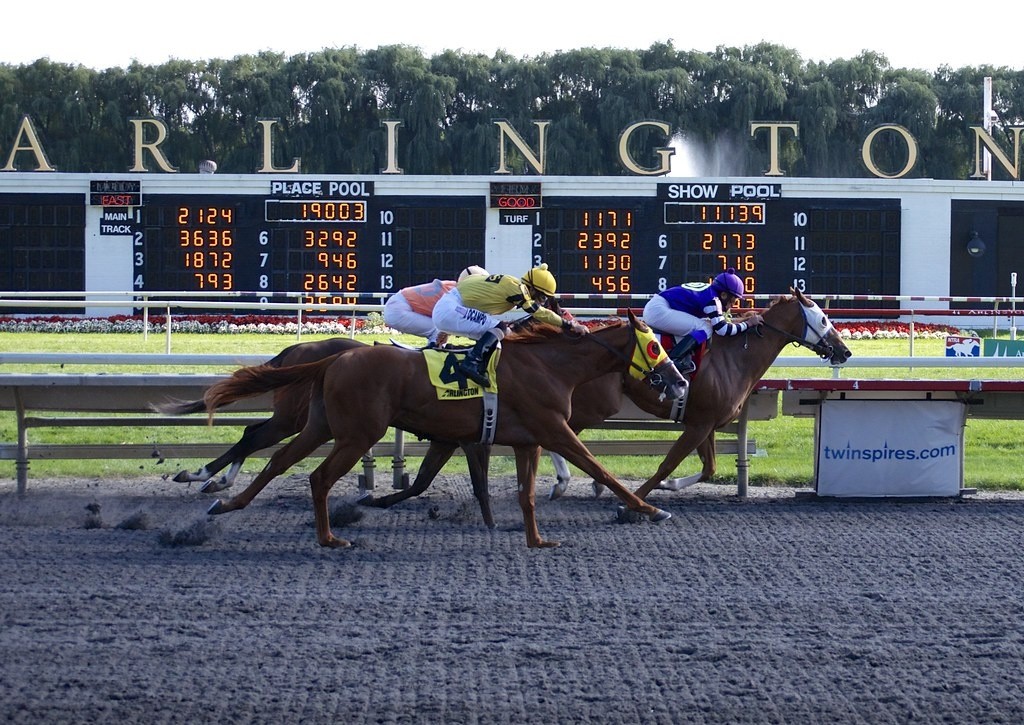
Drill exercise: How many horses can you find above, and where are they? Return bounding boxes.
[142,286,853,550]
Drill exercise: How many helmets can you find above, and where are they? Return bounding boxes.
[458,265,490,282]
[712,267,746,301]
[520,262,557,297]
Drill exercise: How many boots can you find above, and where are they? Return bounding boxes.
[668,334,699,375]
[457,330,499,388]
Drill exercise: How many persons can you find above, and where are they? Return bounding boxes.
[643,269,763,368]
[384,266,490,348]
[433,264,591,387]
